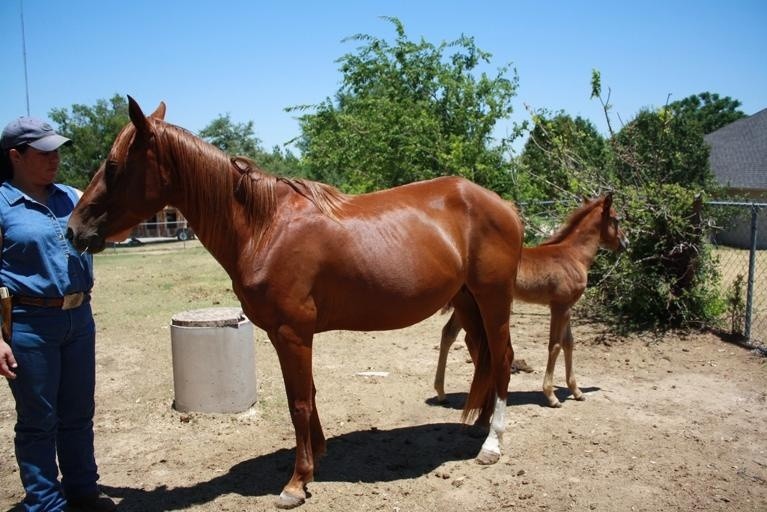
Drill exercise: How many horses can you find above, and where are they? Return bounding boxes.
[63,92,527,509]
[431,189,631,410]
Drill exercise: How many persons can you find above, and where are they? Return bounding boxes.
[0,117,134,512]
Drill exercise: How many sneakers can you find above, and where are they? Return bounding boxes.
[71,497,116,511]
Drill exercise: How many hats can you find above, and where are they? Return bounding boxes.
[0,116,74,154]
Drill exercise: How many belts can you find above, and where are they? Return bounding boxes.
[16,294,90,310]
